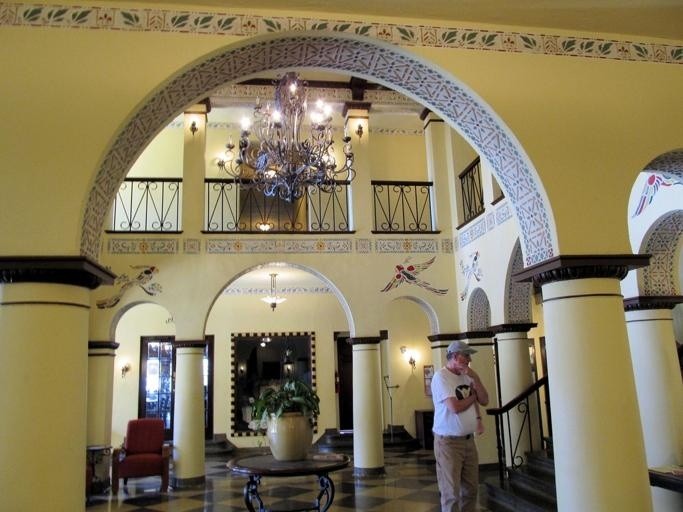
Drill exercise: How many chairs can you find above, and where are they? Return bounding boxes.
[112,418,168,495]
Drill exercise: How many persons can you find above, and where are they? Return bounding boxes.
[474,400,484,436]
[431,340,490,512]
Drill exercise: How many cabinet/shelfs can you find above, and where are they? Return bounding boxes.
[414,410,433,450]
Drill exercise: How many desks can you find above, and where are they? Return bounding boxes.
[225,452,350,512]
[86,446,111,497]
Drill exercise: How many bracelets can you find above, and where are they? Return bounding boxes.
[476,417,481,421]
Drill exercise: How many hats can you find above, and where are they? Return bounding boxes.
[446,340,477,354]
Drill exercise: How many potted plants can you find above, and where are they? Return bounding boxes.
[250,379,320,463]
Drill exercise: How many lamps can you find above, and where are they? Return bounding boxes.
[217,70,355,203]
[259,273,285,312]
